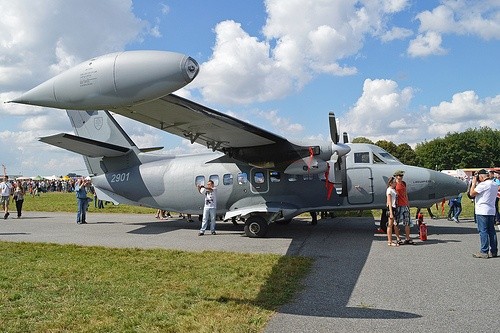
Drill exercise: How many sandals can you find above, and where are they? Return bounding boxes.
[396,239,404,245]
[404,239,417,245]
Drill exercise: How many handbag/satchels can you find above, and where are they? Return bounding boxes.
[13,195,17,200]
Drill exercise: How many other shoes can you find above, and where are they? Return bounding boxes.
[4,212,9,219]
[491,252,497,257]
[186,219,194,223]
[472,251,489,259]
[197,232,204,236]
[388,242,397,247]
[448,217,454,221]
[452,216,459,223]
[212,232,216,235]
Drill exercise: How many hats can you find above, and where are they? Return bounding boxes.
[478,169,489,174]
[392,169,406,176]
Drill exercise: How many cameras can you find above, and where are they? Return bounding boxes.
[200,185,204,188]
[474,174,479,180]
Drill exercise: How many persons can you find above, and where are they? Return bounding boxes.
[155,209,195,222]
[12,180,25,218]
[416,206,436,219]
[386,171,417,246]
[470,169,498,259]
[447,193,462,222]
[467,170,500,231]
[0,178,94,198]
[197,180,217,236]
[75,176,89,224]
[0,176,12,219]
[94,193,110,209]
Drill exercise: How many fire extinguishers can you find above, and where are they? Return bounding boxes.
[418,212,423,229]
[420,222,427,241]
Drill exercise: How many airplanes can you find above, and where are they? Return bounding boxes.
[3,49,469,239]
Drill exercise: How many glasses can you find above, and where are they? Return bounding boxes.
[399,174,403,177]
